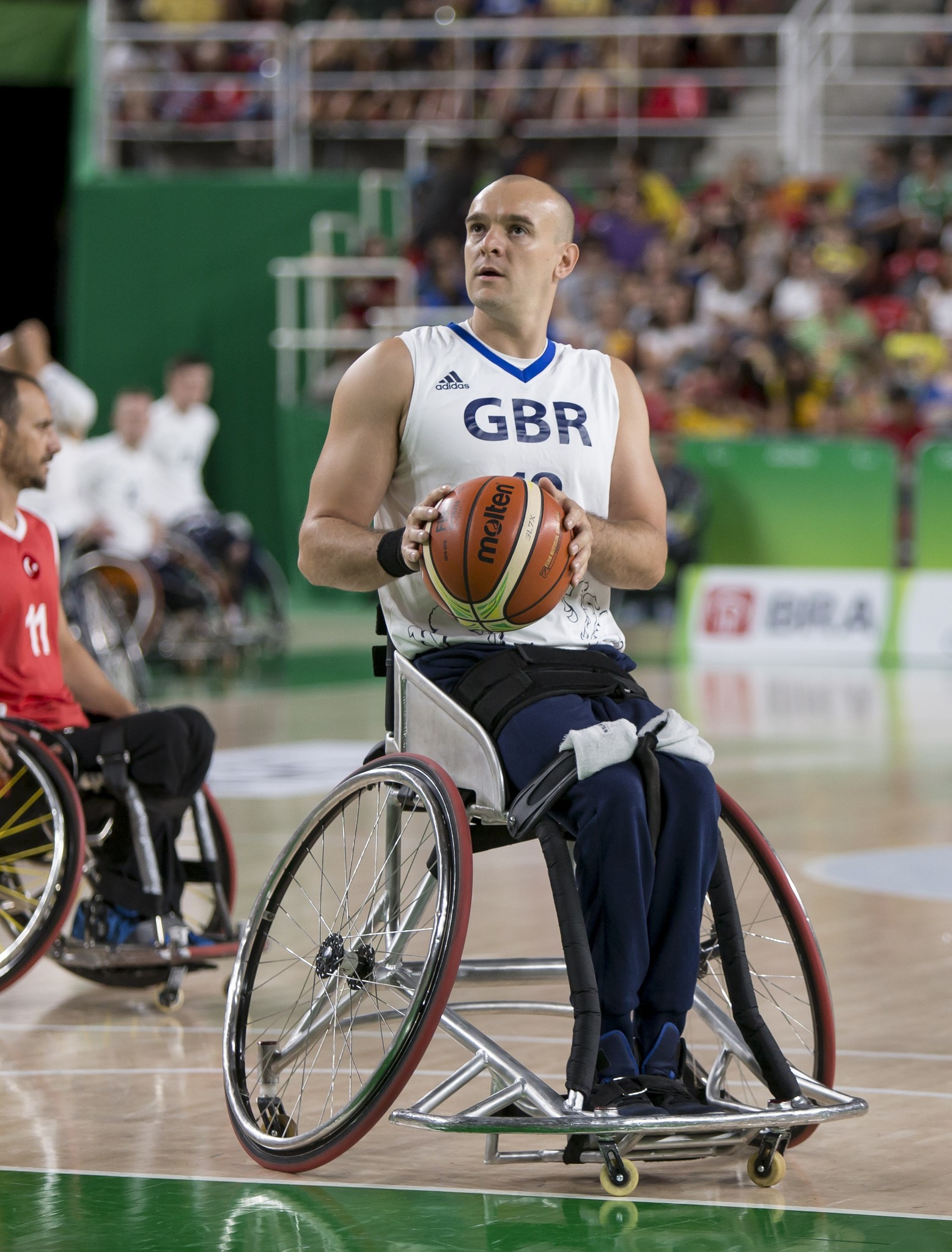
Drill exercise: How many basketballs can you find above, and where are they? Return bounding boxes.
[422,473,575,635]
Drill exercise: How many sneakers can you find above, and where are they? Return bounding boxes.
[639,1022,727,1116]
[584,1029,670,1119]
[69,898,214,948]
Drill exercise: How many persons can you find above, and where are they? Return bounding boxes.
[0,321,100,441]
[84,392,251,664]
[621,439,703,631]
[19,429,84,625]
[114,1,952,439]
[148,357,266,647]
[298,174,760,1142]
[0,368,216,951]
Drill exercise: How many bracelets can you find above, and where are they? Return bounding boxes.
[377,527,419,578]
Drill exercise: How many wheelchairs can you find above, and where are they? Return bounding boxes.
[0,711,246,1001]
[58,515,300,690]
[220,747,866,1197]
